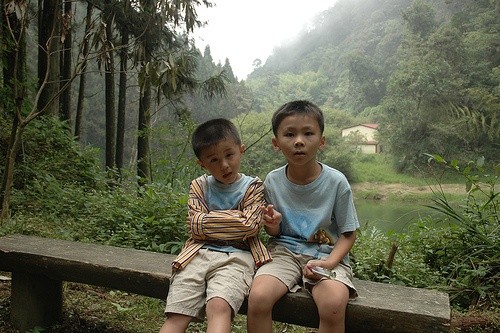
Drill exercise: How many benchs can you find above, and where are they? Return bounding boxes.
[0,232,452,332]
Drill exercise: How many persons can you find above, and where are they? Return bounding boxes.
[247,99,360,333]
[156,117,268,333]
